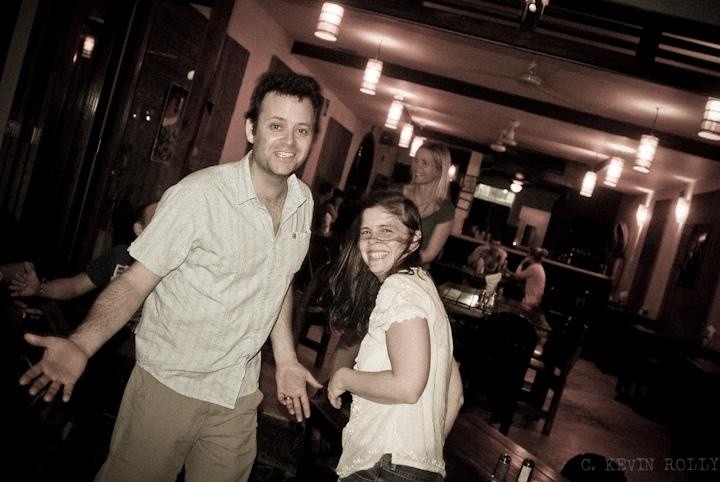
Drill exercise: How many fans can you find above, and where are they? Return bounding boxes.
[489,126,509,155]
[439,59,576,104]
[482,121,547,156]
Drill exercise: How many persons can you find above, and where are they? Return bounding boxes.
[19,70,324,482]
[9,202,157,359]
[327,190,464,482]
[513,246,549,310]
[467,235,508,273]
[327,141,455,376]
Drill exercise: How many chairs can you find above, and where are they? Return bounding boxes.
[293,260,332,368]
[461,306,541,438]
[519,308,589,455]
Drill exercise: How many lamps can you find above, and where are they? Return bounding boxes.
[81,35,95,58]
[580,94,720,198]
[312,1,428,159]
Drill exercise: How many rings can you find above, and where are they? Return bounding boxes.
[284,396,293,400]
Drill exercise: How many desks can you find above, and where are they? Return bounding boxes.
[456,259,512,288]
[584,300,719,475]
[303,399,571,482]
[438,282,553,331]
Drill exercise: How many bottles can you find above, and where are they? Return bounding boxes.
[489,454,535,482]
